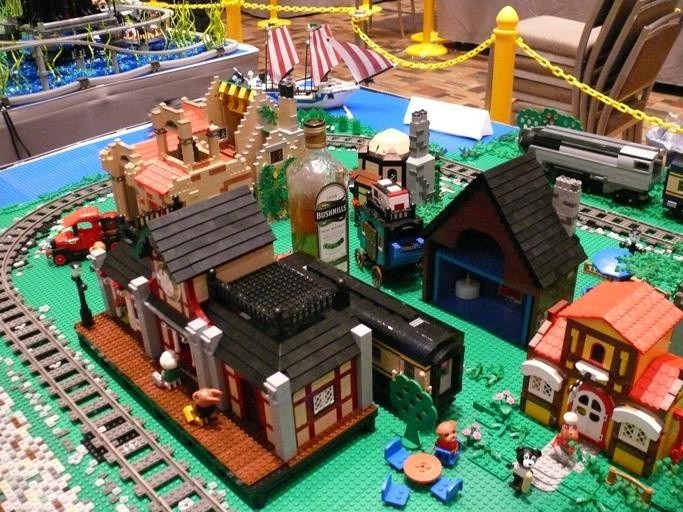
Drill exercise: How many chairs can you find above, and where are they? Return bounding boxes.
[485,1,682,146]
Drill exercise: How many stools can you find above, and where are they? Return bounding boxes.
[367,0,416,40]
